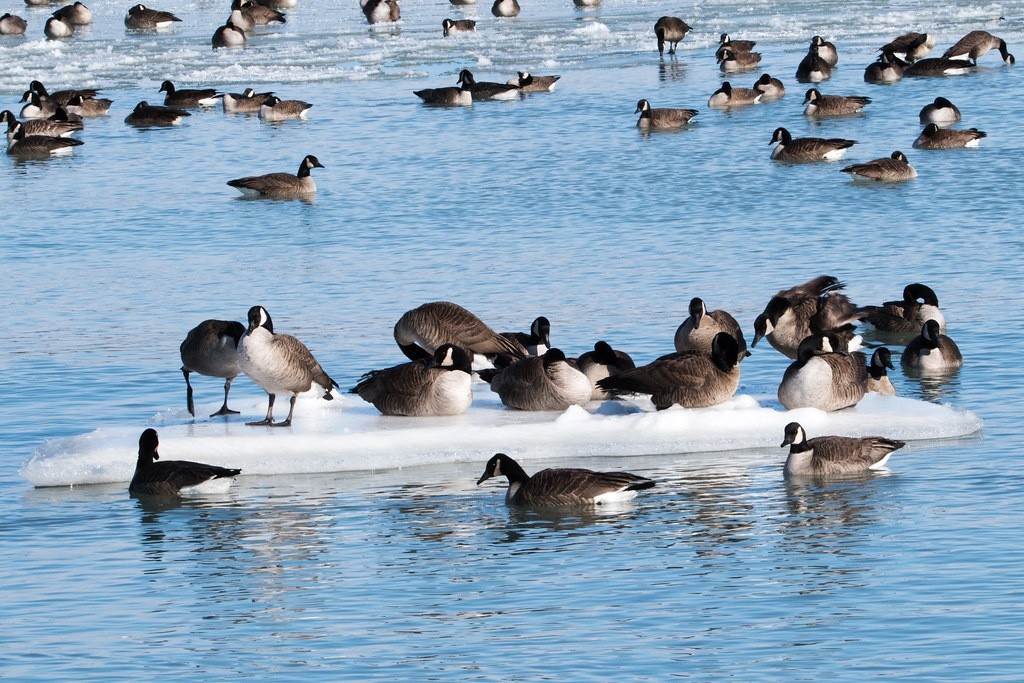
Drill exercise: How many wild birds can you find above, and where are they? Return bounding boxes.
[913,96,987,148]
[767,126,856,163]
[129,428,243,495]
[475,452,657,506]
[0,0,298,48]
[227,153,325,198]
[179,319,247,417]
[633,100,699,126]
[125,80,314,128]
[803,88,874,117]
[714,32,762,73]
[245,306,338,412]
[358,0,600,36]
[795,35,839,81]
[707,73,786,105]
[653,16,694,54]
[864,29,1016,82]
[779,421,908,476]
[347,273,962,419]
[0,80,115,155]
[839,150,918,182]
[413,67,561,105]
